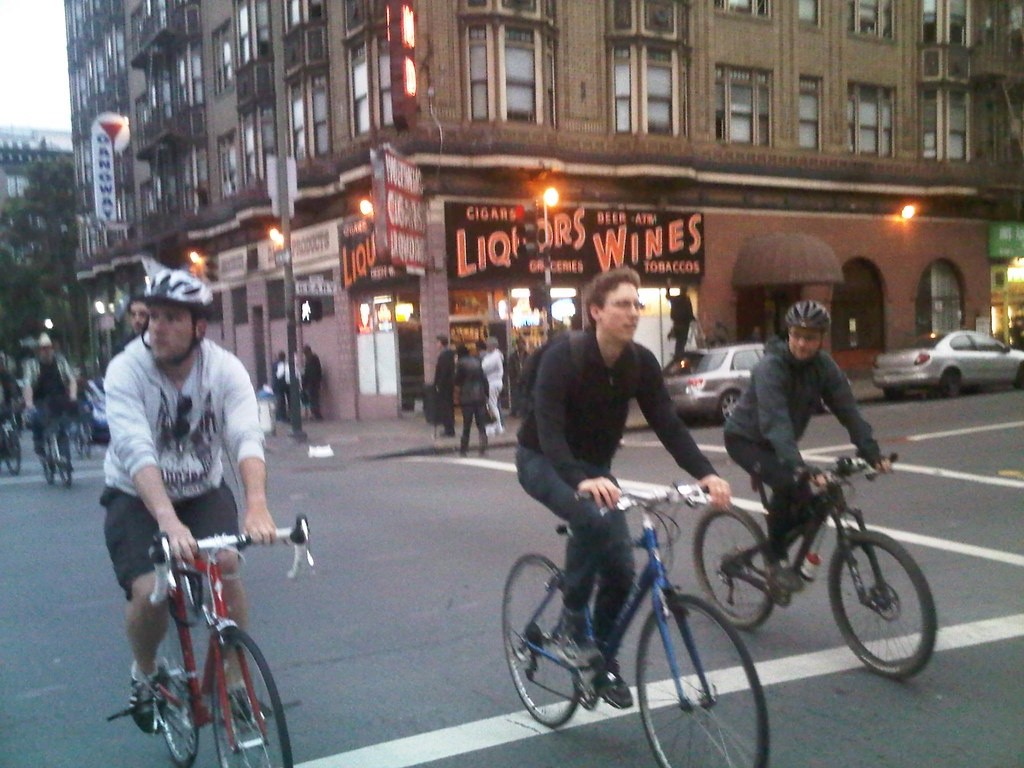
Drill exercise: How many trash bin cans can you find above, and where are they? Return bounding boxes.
[256,384,278,437]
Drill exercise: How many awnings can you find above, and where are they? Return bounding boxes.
[733,231,844,286]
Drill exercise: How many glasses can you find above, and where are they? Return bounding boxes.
[601,299,646,313]
[174,393,192,439]
[789,332,823,342]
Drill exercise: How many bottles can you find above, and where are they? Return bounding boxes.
[799,552,819,583]
[512,320,543,351]
[451,325,489,356]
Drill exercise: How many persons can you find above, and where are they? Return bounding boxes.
[98,270,292,749]
[475,336,529,434]
[0,293,150,470]
[513,267,732,709]
[452,345,488,457]
[665,279,697,359]
[270,344,324,422]
[724,301,892,592]
[434,333,455,438]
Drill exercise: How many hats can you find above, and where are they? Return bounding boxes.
[37,334,53,346]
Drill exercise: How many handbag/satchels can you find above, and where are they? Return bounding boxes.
[486,400,498,425]
[279,376,288,392]
[301,389,310,406]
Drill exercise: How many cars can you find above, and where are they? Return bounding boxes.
[82,378,110,443]
[872,329,1024,396]
[658,340,768,424]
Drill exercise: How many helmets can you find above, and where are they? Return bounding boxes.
[785,300,830,330]
[144,268,214,305]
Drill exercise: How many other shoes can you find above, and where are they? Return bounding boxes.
[772,558,803,592]
[553,622,605,670]
[603,670,634,710]
[34,436,44,456]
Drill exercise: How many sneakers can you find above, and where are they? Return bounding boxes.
[131,663,167,736]
[229,686,271,723]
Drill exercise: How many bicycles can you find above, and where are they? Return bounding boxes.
[1,399,96,487]
[150,515,315,768]
[692,446,941,679]
[499,480,772,767]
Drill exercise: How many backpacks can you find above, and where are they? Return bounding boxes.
[516,330,640,419]
